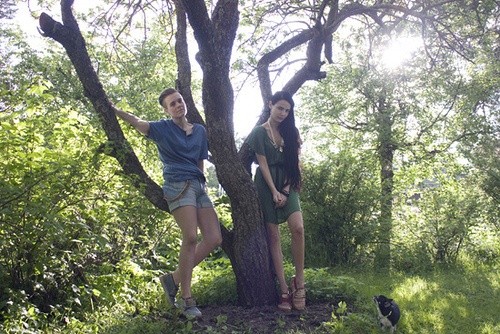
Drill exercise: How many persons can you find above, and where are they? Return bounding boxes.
[246,91,305,312]
[111,87,223,322]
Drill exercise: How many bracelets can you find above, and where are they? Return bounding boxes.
[281,189,290,198]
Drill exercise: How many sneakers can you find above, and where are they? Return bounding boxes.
[177,296,200,319]
[278,293,291,309]
[159,274,177,307]
[289,277,307,308]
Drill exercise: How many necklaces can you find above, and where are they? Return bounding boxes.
[267,118,284,153]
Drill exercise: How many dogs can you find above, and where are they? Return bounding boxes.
[373,295,400,334]
